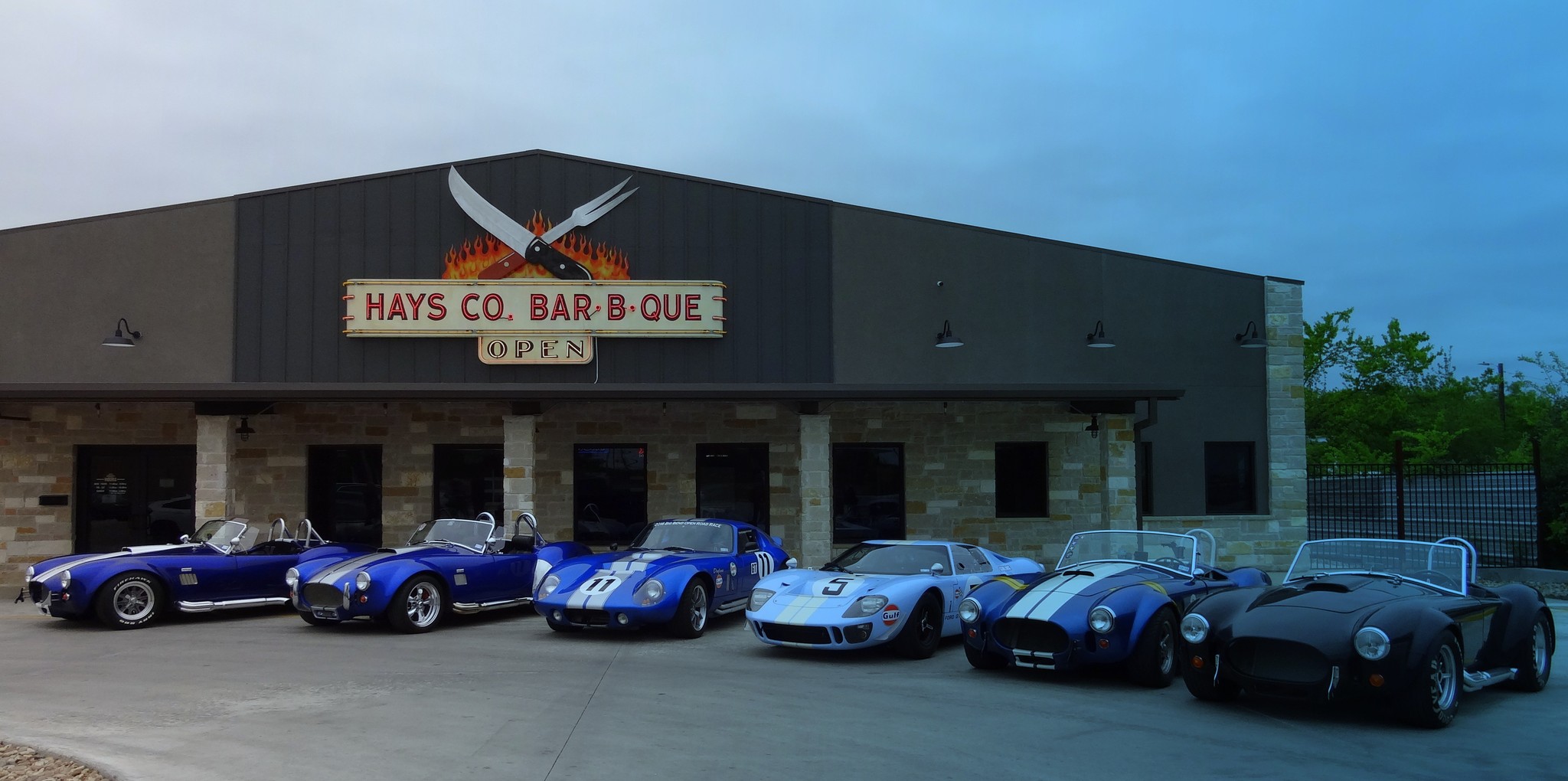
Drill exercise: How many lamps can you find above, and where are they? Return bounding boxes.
[936,319,963,348]
[235,415,255,442]
[1085,414,1100,439]
[1234,321,1269,348]
[103,318,142,346]
[1087,320,1115,348]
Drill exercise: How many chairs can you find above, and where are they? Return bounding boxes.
[511,511,536,555]
[1428,537,1478,596]
[1179,529,1219,580]
[252,517,312,556]
[476,513,494,537]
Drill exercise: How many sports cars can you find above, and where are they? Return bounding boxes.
[744,539,1046,661]
[13,516,340,632]
[1178,536,1556,730]
[286,509,554,636]
[532,518,794,641]
[957,527,1273,690]
